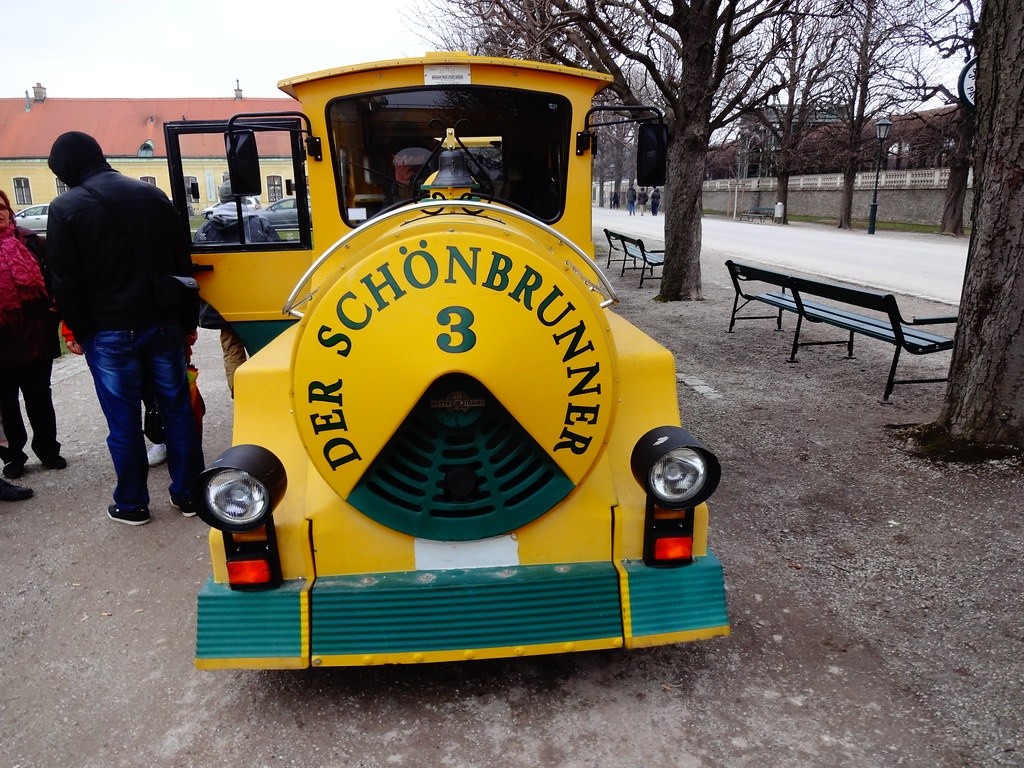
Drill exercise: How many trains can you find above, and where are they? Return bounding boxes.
[161,49,733,669]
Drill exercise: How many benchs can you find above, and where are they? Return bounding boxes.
[602,228,665,290]
[724,259,959,405]
[740,207,775,223]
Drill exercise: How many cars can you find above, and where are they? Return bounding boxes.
[201,197,262,220]
[170,200,194,216]
[14,204,47,231]
[258,197,313,229]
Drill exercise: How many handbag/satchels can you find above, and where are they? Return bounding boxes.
[142,367,169,443]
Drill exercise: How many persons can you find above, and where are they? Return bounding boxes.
[46,130,205,525]
[1,190,67,502]
[627,184,636,215]
[638,188,648,216]
[649,186,660,216]
[613,192,619,209]
[194,182,281,398]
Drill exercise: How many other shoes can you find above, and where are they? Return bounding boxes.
[31,442,67,469]
[2,458,24,478]
[0,478,34,500]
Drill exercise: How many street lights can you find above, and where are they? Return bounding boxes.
[867,117,894,234]
[608,165,615,208]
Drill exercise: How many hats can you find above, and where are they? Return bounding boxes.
[219,180,243,204]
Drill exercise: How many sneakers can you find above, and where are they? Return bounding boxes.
[169,497,196,516]
[146,444,167,464]
[106,505,152,525]
